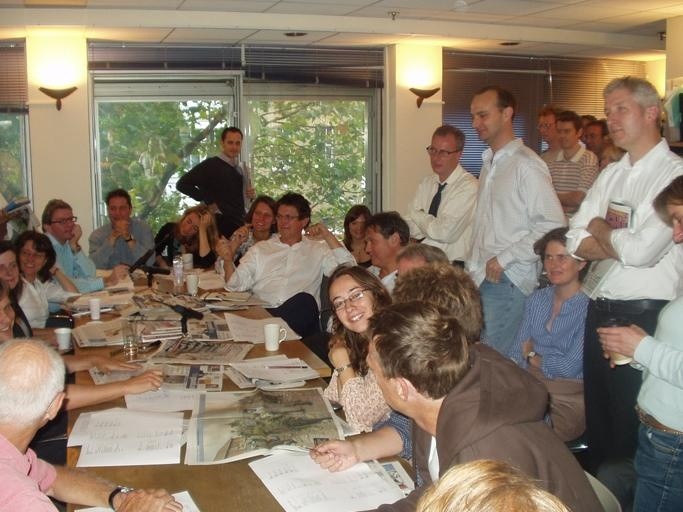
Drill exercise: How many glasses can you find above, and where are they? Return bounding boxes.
[426,146,458,158]
[276,214,297,223]
[332,289,370,313]
[52,216,78,224]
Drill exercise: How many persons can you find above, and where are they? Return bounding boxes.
[309,75,683,511]
[0,339,183,511]
[1,279,164,507]
[176,126,255,238]
[152,204,223,271]
[216,191,356,343]
[0,188,164,464]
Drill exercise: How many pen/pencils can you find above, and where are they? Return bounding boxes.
[264,365,307,369]
[297,445,323,457]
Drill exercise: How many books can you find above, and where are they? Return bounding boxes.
[67,275,346,465]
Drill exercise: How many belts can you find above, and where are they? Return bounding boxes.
[594,296,667,317]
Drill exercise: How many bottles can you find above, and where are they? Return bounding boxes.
[123,334,138,363]
[172,254,184,288]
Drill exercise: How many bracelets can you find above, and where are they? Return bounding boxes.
[108,485,134,510]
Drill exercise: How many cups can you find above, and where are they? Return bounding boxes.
[183,254,193,274]
[260,324,286,352]
[598,317,634,368]
[88,297,100,320]
[185,273,200,295]
[53,327,72,351]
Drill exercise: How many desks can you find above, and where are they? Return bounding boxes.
[67,261,413,512]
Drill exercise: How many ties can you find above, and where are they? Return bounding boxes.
[427,183,447,218]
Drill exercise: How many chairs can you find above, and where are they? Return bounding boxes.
[565,431,602,454]
[584,469,623,512]
